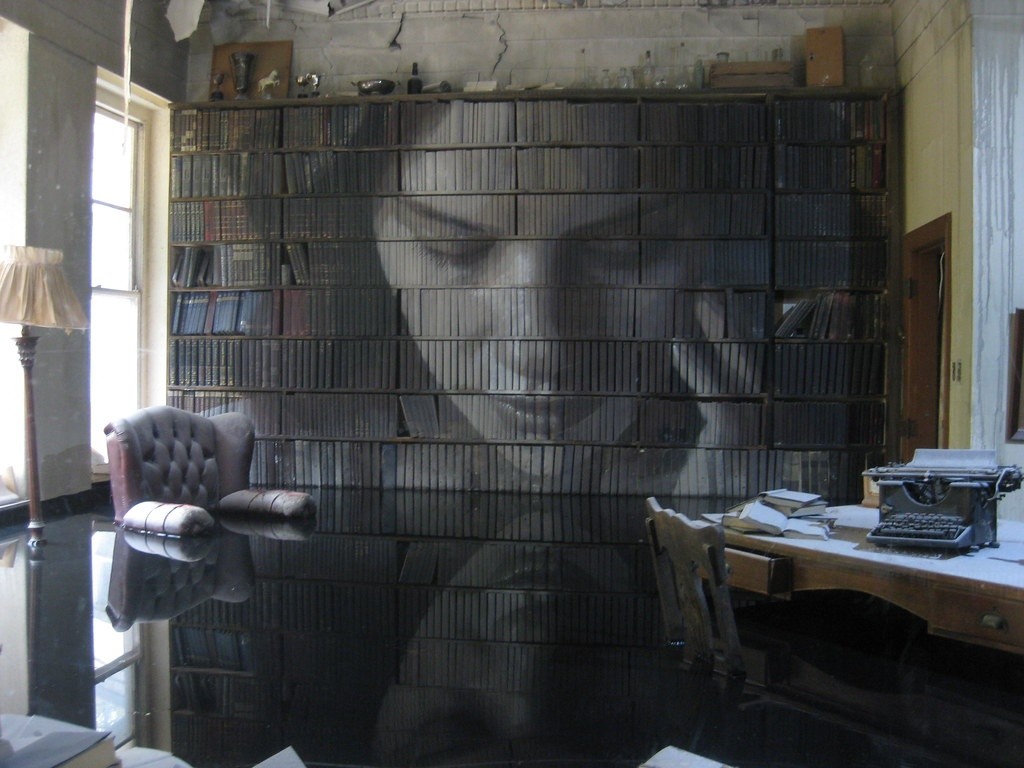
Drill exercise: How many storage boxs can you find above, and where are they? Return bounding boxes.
[806,25,845,87]
[708,61,799,89]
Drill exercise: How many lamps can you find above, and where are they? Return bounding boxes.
[0,244,88,549]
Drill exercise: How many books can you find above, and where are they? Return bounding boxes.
[165,502,735,765]
[171,94,894,500]
[702,487,837,541]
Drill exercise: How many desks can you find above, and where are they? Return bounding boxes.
[696,506,1023,653]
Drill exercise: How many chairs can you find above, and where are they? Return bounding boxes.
[645,496,756,681]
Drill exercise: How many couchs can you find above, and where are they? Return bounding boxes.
[104,405,318,537]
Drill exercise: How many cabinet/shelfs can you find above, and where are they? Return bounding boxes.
[165,88,899,496]
